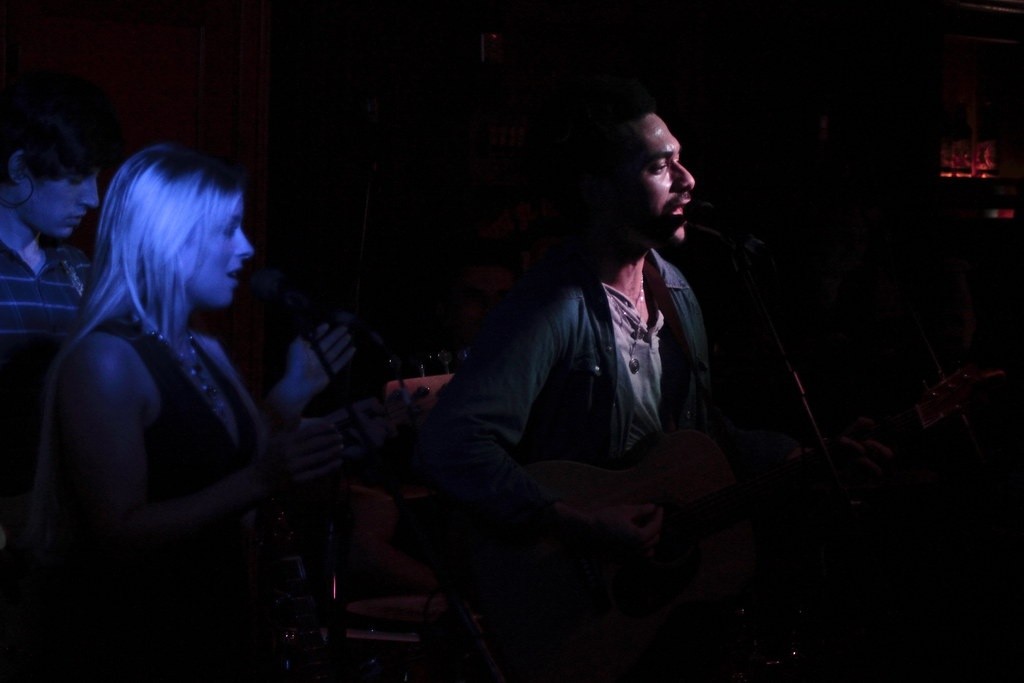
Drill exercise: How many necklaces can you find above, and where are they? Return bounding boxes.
[139,323,229,424]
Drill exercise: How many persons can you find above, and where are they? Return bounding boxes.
[413,91,894,683]
[1,66,408,683]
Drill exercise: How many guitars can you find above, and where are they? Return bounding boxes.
[434,369,1009,683]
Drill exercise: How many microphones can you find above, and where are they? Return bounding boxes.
[683,201,766,252]
[248,269,362,329]
